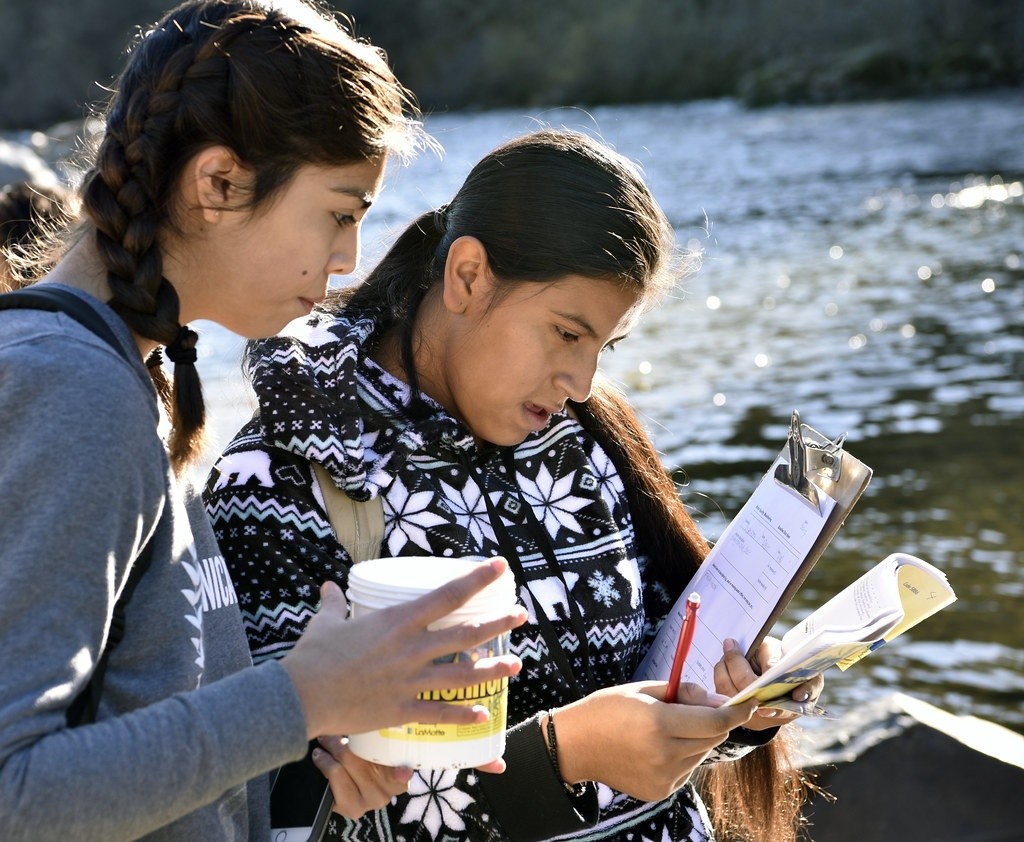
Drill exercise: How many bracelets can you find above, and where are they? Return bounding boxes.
[546,708,586,797]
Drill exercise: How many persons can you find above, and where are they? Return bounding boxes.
[0,0,529,842]
[203,131,824,842]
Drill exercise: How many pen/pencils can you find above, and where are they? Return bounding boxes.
[667,593,699,704]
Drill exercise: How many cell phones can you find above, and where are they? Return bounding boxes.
[270,739,336,842]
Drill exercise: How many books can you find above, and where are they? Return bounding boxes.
[718,552,957,708]
